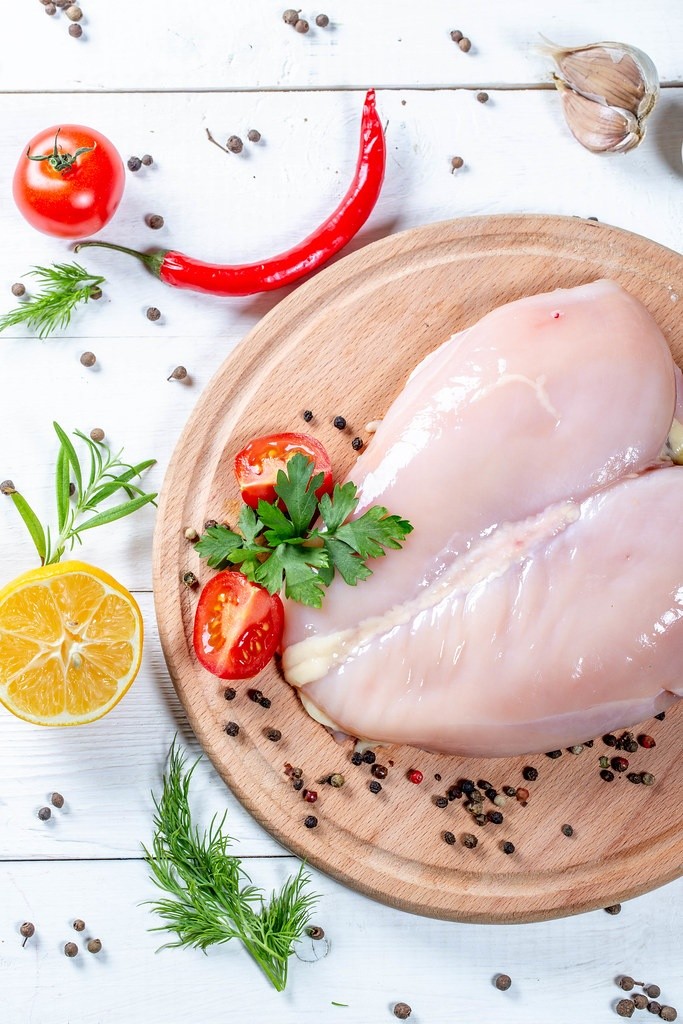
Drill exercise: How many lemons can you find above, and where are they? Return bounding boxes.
[1,561,144,727]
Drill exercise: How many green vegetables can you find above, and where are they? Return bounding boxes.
[193,453,414,607]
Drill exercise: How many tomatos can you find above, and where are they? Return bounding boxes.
[194,571,284,680]
[231,433,334,510]
[11,125,130,242]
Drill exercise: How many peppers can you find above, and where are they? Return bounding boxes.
[72,89,386,297]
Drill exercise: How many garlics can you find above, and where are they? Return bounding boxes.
[529,28,659,154]
